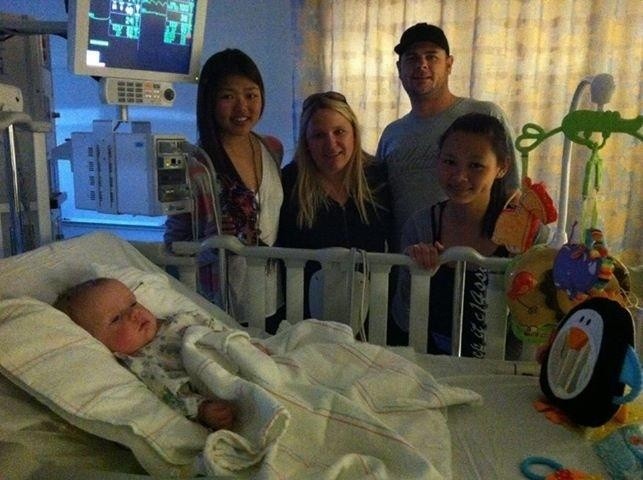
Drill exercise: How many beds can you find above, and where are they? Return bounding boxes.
[1,237,643,479]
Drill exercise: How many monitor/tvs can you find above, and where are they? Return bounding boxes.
[69,0,207,84]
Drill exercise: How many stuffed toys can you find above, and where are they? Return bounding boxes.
[592,424,643,480]
[531,296,643,428]
[491,177,558,254]
[505,232,576,345]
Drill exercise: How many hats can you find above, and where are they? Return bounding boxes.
[394,24,451,58]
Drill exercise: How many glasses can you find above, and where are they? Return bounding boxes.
[300,92,349,118]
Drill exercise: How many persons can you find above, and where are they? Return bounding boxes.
[552,228,643,316]
[391,112,555,361]
[278,92,399,347]
[375,23,520,347]
[164,48,287,335]
[52,278,233,430]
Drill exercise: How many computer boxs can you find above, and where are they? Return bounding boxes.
[67,122,199,222]
